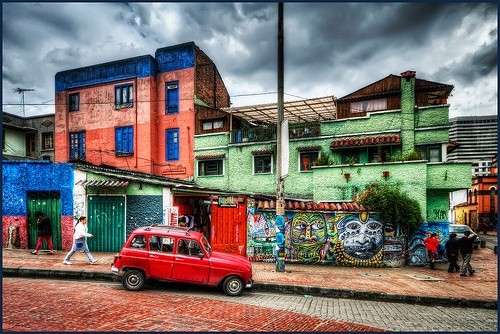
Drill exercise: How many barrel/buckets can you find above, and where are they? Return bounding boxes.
[483,229,487,235]
[494,246,498,254]
[481,241,486,248]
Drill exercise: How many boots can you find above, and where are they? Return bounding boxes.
[430,262,437,269]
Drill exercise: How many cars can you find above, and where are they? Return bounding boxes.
[449,224,480,250]
[111,223,254,296]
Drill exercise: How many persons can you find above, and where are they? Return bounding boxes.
[445,233,460,273]
[31,211,53,254]
[459,230,477,275]
[63,216,98,264]
[424,233,439,269]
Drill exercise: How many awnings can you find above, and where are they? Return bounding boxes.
[75,180,129,193]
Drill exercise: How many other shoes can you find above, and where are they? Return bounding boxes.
[470,270,475,275]
[29,251,37,254]
[63,260,72,265]
[460,273,467,276]
[455,266,460,272]
[46,252,54,255]
[90,258,99,265]
[448,270,455,273]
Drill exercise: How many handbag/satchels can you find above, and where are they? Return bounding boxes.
[75,241,84,250]
